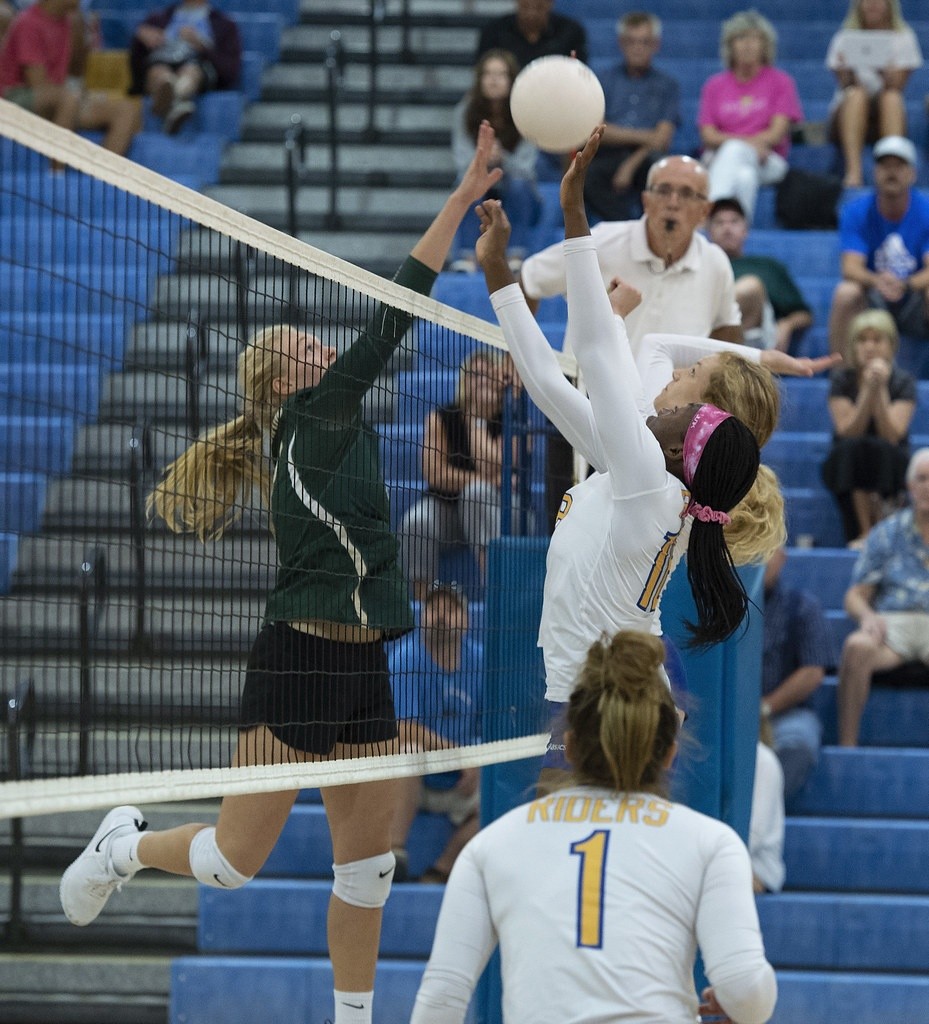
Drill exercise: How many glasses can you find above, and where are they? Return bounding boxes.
[651,186,707,203]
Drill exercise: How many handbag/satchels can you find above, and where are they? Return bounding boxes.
[773,168,843,232]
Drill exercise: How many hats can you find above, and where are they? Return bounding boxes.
[710,195,745,218]
[873,135,919,165]
[423,578,470,608]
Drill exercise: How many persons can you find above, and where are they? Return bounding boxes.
[60,117,504,1024]
[476,124,843,801]
[0,0,242,175]
[387,579,482,885]
[397,0,815,604]
[820,0,929,751]
[750,548,833,893]
[409,628,777,1024]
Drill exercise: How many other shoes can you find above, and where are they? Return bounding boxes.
[389,844,410,882]
[509,258,523,272]
[155,80,197,136]
[453,260,476,273]
[419,868,448,885]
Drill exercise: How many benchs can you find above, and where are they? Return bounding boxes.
[0,0,929,1024]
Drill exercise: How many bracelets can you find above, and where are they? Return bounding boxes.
[760,699,774,718]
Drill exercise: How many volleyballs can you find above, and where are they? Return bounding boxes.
[509,55,606,155]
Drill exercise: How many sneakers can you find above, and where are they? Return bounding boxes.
[61,803,147,926]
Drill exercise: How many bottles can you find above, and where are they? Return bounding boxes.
[894,257,909,280]
